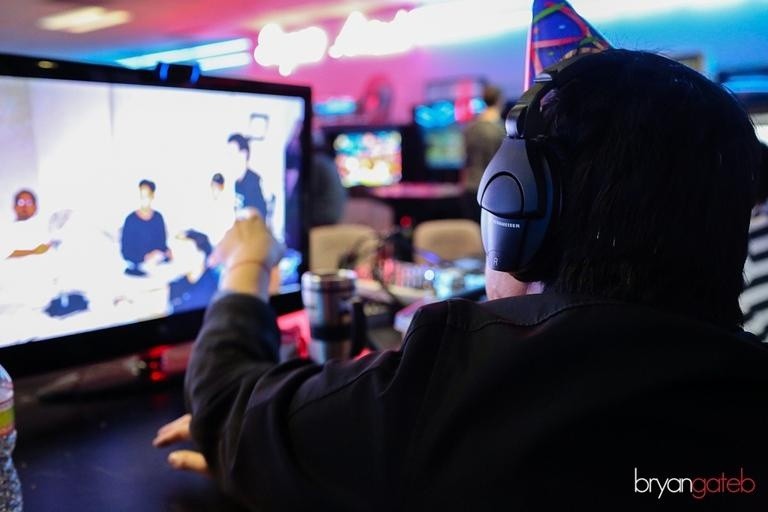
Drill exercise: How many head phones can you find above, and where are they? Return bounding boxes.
[476,54,595,282]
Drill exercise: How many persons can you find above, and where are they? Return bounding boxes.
[152,52,767,511]
[0,132,268,317]
[309,130,344,227]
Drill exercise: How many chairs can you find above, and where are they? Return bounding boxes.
[309,225,376,285]
[414,219,482,269]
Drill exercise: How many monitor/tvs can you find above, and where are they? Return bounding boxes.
[330,124,406,189]
[0,50,315,400]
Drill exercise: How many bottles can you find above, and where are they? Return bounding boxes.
[0,364,28,511]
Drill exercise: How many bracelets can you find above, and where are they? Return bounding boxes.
[223,260,270,278]
